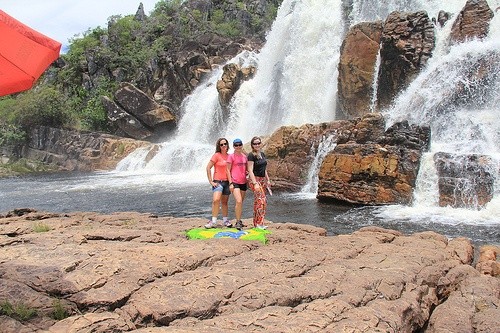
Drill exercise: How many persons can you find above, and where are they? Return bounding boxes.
[226,139,248,231]
[204,138,232,229]
[247,136,272,230]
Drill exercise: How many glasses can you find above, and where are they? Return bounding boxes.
[252,143,260,145]
[234,144,242,147]
[220,143,227,146]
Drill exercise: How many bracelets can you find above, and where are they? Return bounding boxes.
[229,182,232,185]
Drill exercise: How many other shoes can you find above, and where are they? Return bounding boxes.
[236,222,241,229]
[224,221,232,226]
[204,223,217,228]
[258,225,268,229]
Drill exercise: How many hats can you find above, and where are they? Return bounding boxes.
[233,139,242,144]
